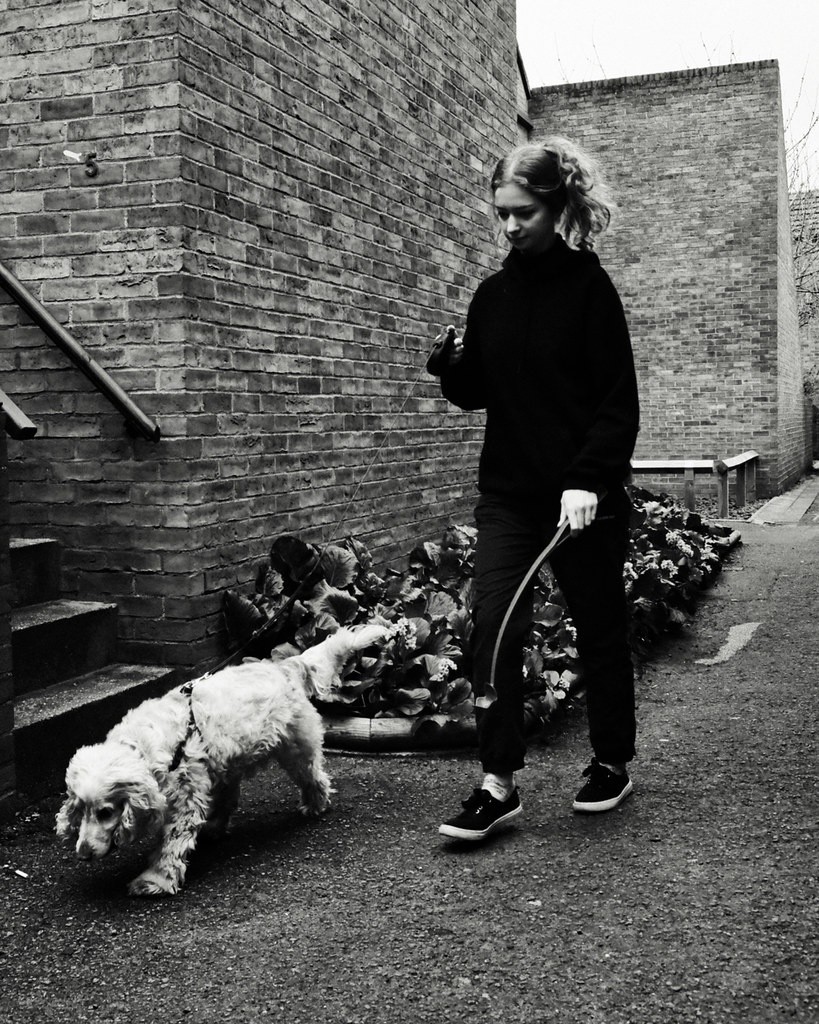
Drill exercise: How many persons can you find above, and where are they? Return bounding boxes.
[426,134,642,841]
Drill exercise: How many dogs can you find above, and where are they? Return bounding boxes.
[54,622,388,895]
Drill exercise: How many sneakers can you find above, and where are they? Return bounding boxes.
[438,788,522,841]
[573,757,632,811]
